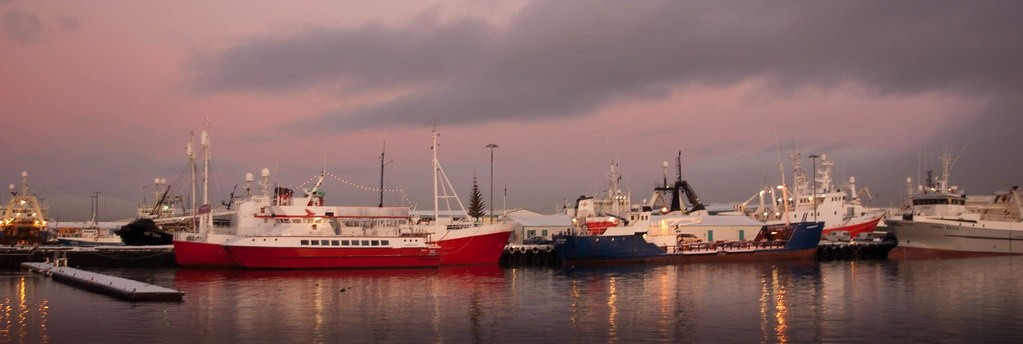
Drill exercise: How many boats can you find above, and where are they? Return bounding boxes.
[0,128,1023,271]
